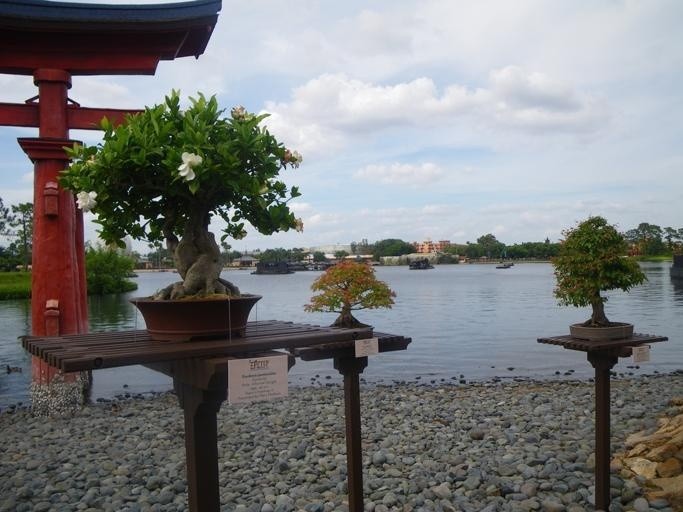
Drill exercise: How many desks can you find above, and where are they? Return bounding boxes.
[537,332,668,511]
[21,320,411,512]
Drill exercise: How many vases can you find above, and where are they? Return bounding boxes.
[129,295,262,342]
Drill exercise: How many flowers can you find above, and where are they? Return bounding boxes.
[55,89,305,299]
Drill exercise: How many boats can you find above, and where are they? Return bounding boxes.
[251,259,294,275]
[409,257,434,270]
[496,261,513,268]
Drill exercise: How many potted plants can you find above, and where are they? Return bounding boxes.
[301,261,396,338]
[547,215,649,341]
